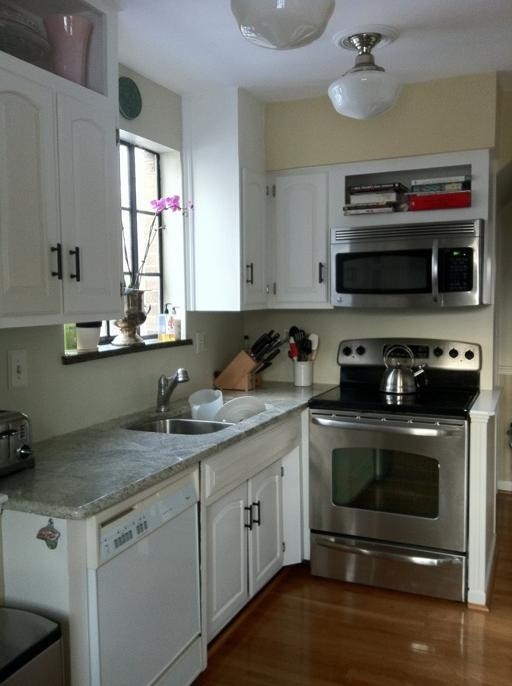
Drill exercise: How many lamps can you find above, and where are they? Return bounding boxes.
[230,1,337,52]
[325,21,403,121]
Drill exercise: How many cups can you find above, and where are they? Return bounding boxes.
[295,362,314,387]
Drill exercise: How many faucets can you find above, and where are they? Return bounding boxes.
[158,367,189,412]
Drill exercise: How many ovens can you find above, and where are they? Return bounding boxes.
[308,408,472,602]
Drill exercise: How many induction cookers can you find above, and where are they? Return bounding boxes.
[309,336,482,420]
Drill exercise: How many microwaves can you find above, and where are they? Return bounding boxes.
[329,219,484,310]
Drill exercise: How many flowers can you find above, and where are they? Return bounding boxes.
[123,192,194,290]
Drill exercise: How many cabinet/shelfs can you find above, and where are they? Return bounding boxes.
[0,2,125,329]
[3,460,209,684]
[202,407,309,650]
[239,148,495,312]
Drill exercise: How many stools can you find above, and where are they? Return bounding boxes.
[0,604,69,685]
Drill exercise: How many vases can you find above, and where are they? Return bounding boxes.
[110,286,152,348]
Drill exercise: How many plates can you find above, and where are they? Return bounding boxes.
[214,395,267,424]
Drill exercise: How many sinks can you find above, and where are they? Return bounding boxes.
[174,401,274,423]
[124,419,235,434]
